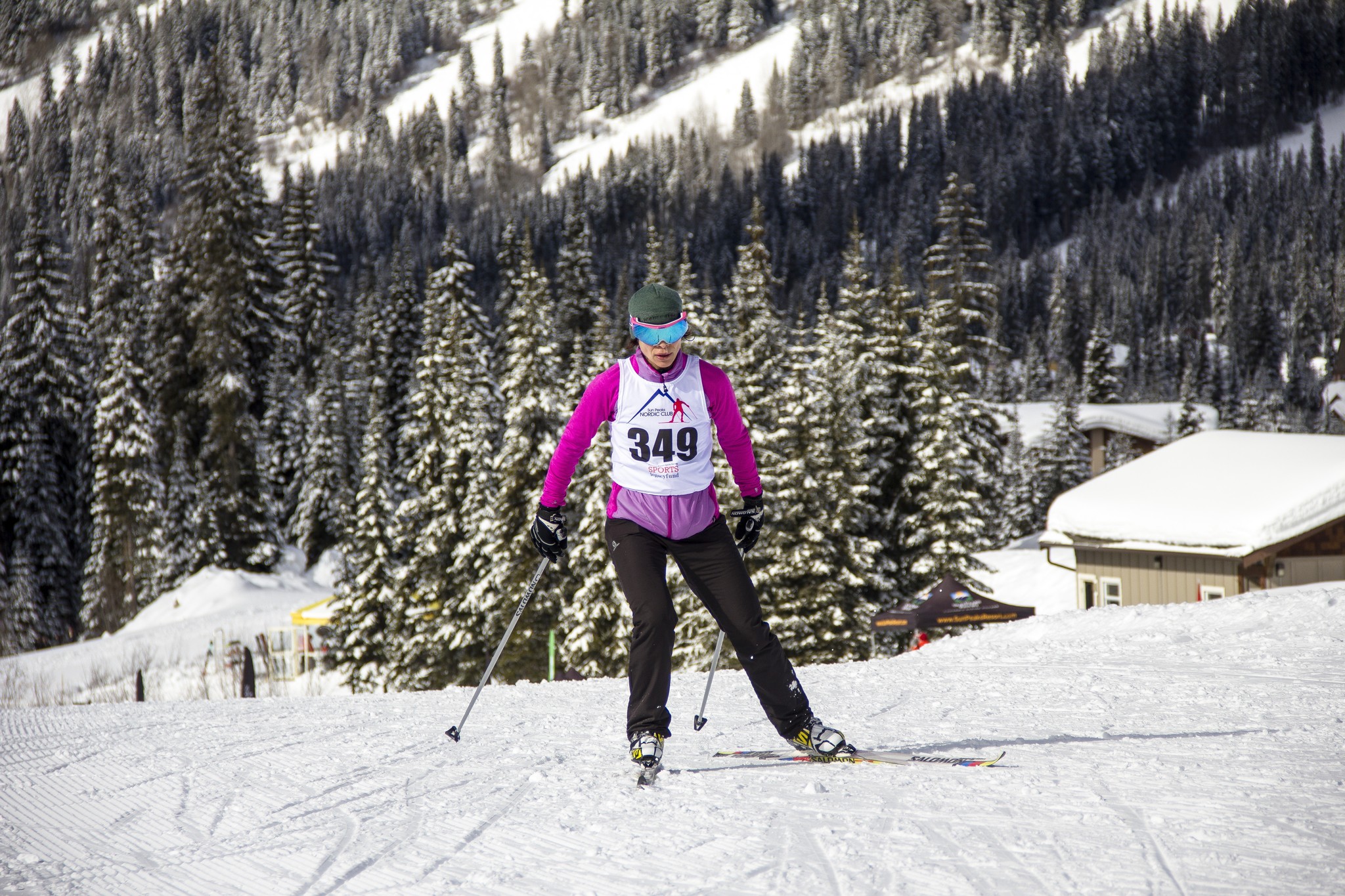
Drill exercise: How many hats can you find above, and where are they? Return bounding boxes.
[629,284,683,325]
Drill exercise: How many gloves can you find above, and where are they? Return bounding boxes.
[730,490,764,553]
[530,502,567,564]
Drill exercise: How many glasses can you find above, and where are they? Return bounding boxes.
[632,311,688,345]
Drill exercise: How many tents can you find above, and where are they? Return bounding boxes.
[871,570,1034,658]
[292,590,444,674]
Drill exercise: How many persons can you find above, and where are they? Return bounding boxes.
[530,284,848,764]
[297,633,317,672]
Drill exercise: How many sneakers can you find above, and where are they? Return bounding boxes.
[629,729,665,762]
[787,716,846,756]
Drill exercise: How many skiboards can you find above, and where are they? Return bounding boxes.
[636,748,1007,787]
